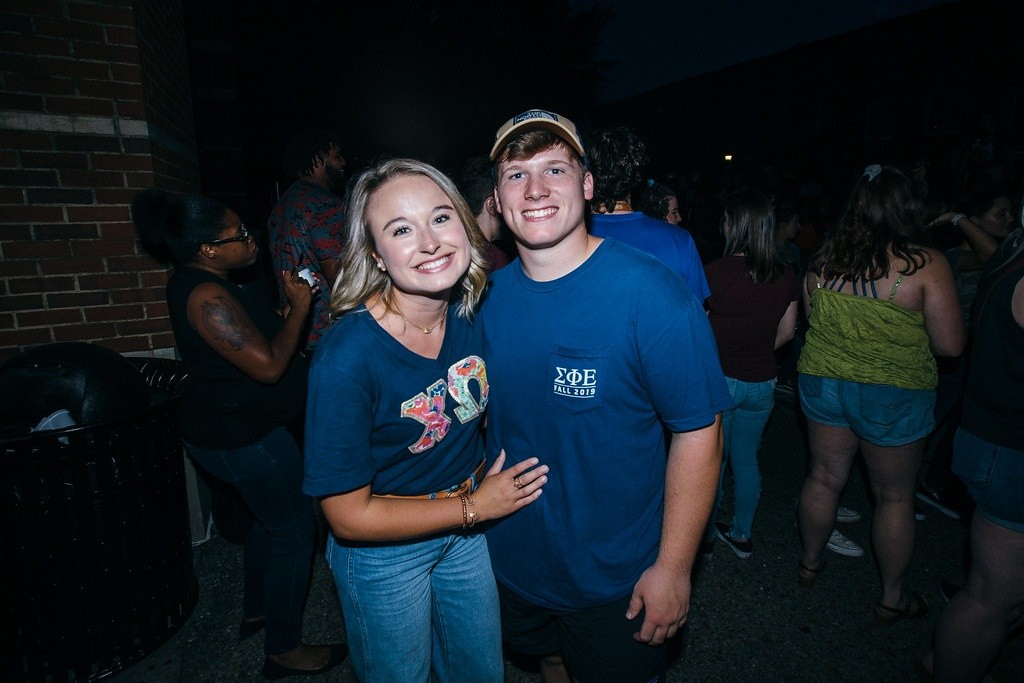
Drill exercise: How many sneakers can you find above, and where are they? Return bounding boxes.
[826,530,864,557]
[837,507,861,522]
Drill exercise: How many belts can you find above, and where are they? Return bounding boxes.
[371,459,486,500]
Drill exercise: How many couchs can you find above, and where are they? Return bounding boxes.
[0,341,201,683]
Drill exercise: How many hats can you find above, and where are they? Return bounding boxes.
[490,109,585,161]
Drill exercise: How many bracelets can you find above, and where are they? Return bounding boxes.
[459,493,480,529]
[950,213,967,223]
[953,214,967,225]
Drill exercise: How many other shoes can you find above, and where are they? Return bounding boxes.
[714,521,753,559]
[263,644,348,680]
[240,612,272,638]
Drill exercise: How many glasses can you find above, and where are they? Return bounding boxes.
[209,223,247,245]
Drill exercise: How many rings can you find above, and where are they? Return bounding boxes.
[513,477,523,489]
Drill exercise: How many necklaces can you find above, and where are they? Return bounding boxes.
[391,305,448,334]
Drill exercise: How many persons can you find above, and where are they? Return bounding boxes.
[265,130,365,555]
[447,122,1024,683]
[132,188,350,680]
[483,108,726,683]
[301,158,549,683]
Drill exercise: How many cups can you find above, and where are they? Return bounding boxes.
[298,268,319,295]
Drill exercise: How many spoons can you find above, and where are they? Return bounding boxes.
[291,252,303,277]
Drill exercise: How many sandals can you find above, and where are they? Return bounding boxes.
[873,591,930,625]
[797,547,831,585]
